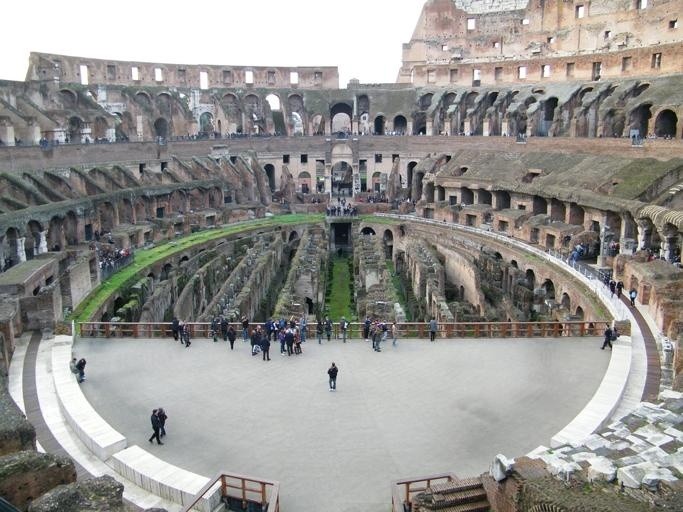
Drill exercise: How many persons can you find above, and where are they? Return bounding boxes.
[308,172,390,218]
[611,326,617,341]
[396,197,416,209]
[68,358,79,381]
[604,322,609,333]
[427,316,438,341]
[165,313,400,362]
[148,408,164,445]
[91,225,130,277]
[565,237,682,309]
[154,407,167,438]
[75,358,86,384]
[326,362,338,393]
[460,201,466,209]
[599,324,612,351]
